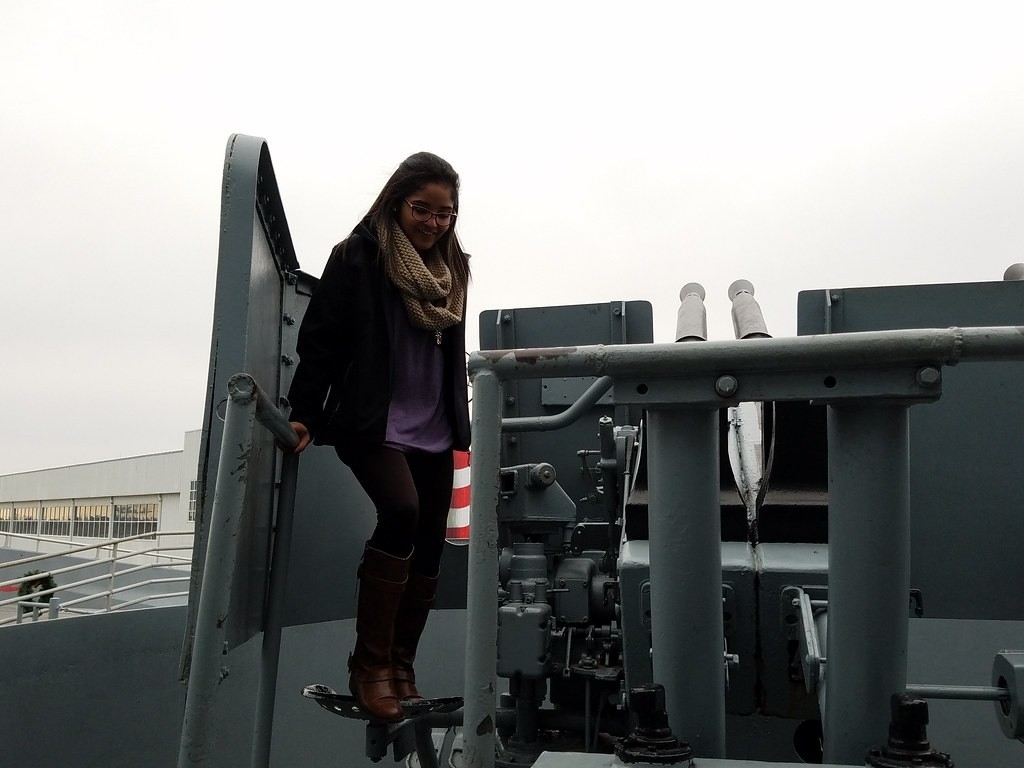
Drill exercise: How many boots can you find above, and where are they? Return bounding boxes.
[348,540,439,722]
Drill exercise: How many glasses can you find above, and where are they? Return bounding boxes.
[403,198,457,226]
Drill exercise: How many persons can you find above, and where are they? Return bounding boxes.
[273,152,472,723]
